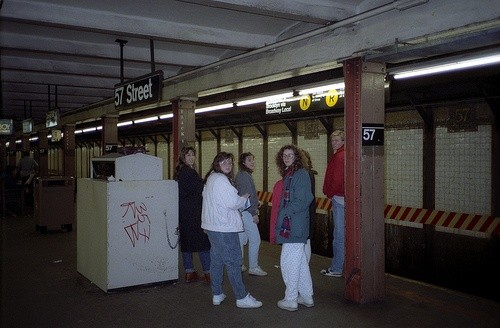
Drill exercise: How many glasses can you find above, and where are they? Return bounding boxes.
[282,153,295,158]
[219,160,233,165]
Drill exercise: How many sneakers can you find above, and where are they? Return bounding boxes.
[296,296,315,307]
[277,298,298,311]
[241,264,247,272]
[236,292,263,308]
[212,294,226,305]
[320,266,342,277]
[248,265,268,276]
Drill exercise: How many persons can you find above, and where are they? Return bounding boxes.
[299,148,318,265]
[320,130,346,278]
[174,147,210,283]
[19,149,39,173]
[234,152,268,276]
[277,145,314,312]
[201,153,263,308]
[270,179,284,246]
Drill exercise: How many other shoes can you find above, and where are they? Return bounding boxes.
[203,273,211,284]
[184,271,202,283]
